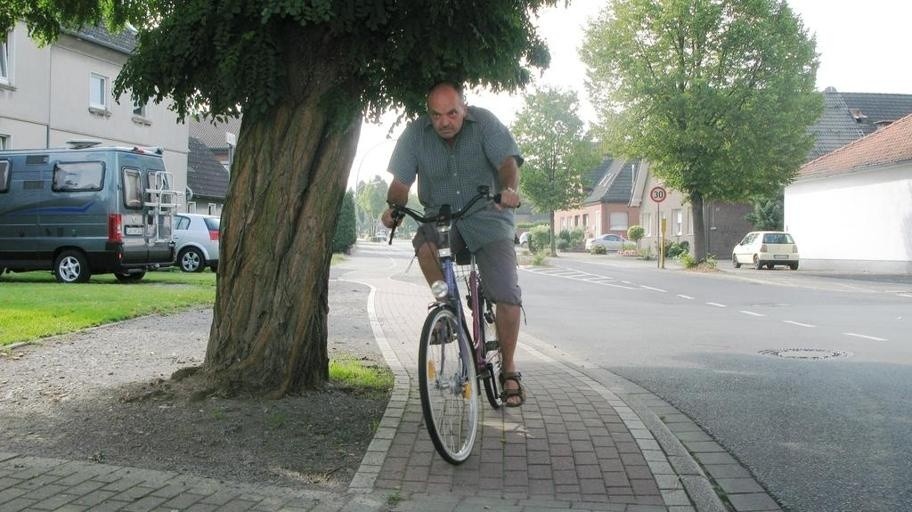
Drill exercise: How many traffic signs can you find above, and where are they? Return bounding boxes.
[651,187,666,202]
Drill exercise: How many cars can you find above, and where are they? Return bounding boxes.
[374,228,388,241]
[586,234,635,253]
[173,214,223,273]
[519,232,532,248]
[734,229,798,270]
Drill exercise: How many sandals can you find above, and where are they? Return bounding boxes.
[499,372,523,407]
[430,318,458,345]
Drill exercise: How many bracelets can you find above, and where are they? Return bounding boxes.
[501,186,516,193]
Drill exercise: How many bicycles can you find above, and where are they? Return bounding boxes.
[386,186,524,464]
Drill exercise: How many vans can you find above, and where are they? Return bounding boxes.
[0,140,180,283]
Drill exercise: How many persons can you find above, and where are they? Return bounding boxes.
[381,78,527,409]
